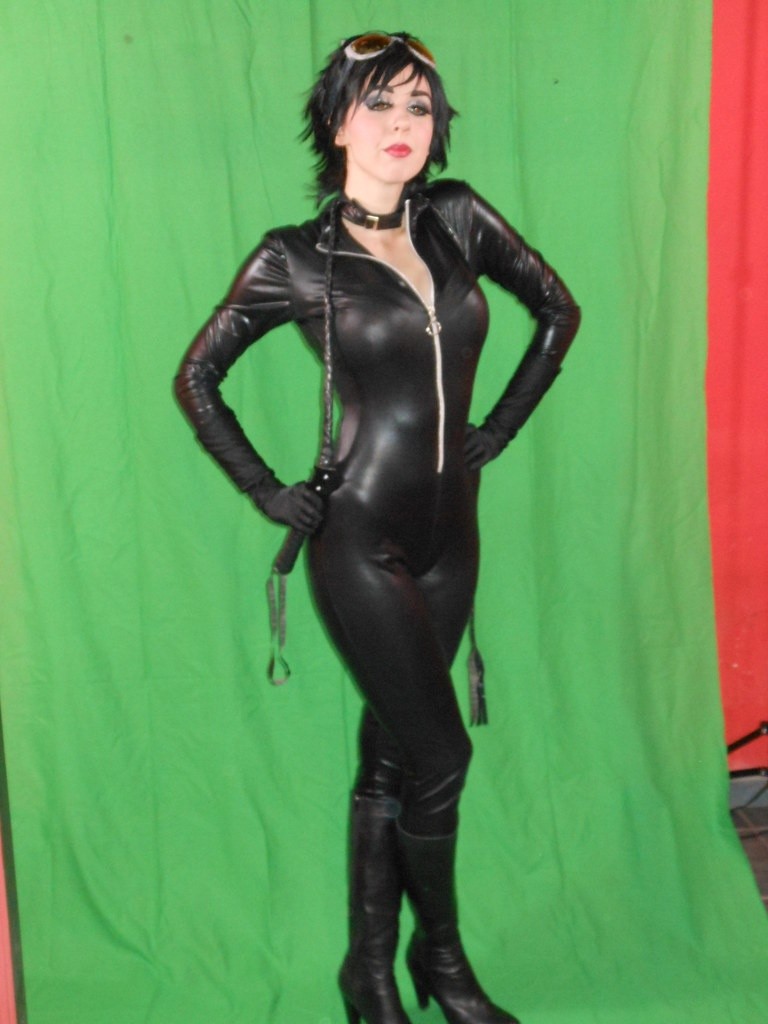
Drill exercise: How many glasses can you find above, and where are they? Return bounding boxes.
[344,31,437,73]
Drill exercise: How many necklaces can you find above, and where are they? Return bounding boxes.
[342,192,403,230]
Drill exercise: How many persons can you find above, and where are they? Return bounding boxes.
[176,30,581,1023]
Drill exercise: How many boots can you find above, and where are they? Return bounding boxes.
[395,817,523,1024]
[340,792,413,1024]
